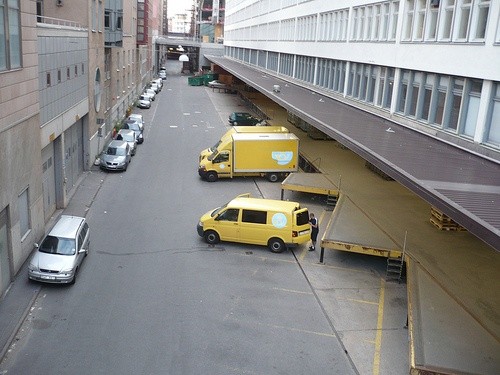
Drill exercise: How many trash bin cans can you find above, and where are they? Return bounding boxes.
[188,73,219,86]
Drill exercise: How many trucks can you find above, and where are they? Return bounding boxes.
[198,126,290,162]
[197,132,300,183]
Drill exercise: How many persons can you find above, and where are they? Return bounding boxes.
[307,213,319,251]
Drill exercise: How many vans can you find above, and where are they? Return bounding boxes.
[197,193,312,253]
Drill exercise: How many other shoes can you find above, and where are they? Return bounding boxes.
[309,246,315,251]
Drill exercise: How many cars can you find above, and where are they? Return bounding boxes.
[116,129,138,156]
[136,95,151,109]
[208,80,225,88]
[228,111,261,127]
[99,140,132,172]
[148,66,167,94]
[128,113,146,131]
[122,120,144,143]
[143,89,157,100]
[26,215,91,286]
